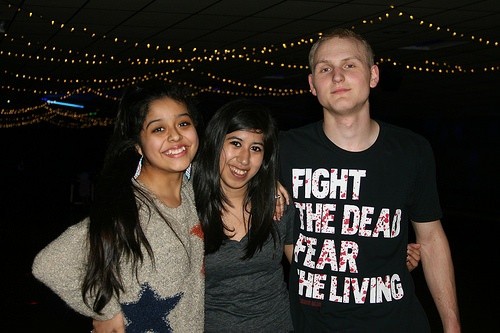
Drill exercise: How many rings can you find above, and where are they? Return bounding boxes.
[273,193,281,199]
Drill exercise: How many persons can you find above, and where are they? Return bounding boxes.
[278,29,461,333]
[193,100,423,333]
[30,78,291,333]
